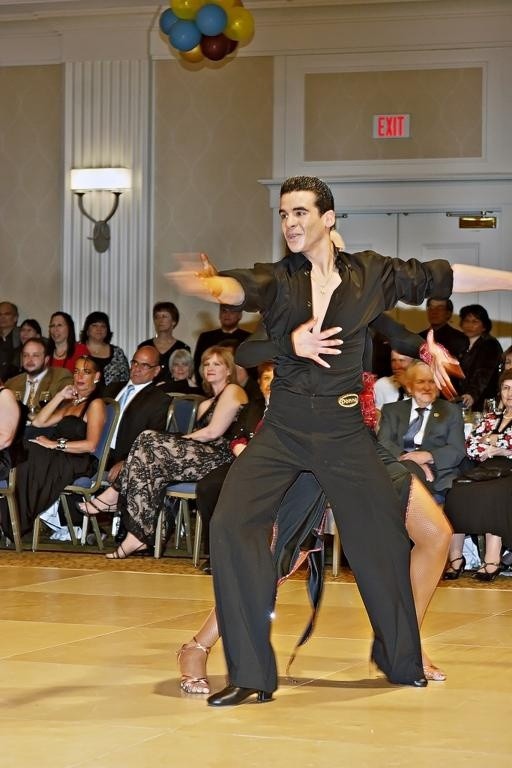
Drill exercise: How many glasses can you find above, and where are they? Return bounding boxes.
[130,359,159,370]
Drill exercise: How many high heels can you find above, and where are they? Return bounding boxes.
[472,559,502,582]
[421,662,447,682]
[443,555,466,580]
[75,496,119,516]
[105,543,148,559]
[176,637,211,698]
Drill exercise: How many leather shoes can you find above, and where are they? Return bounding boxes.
[402,676,428,688]
[206,684,273,707]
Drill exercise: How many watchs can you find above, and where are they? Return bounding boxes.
[55,437,66,452]
[486,435,491,445]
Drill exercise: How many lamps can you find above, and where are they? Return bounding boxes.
[69,166,134,253]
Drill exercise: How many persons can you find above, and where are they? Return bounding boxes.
[502,344,512,369]
[4,336,73,410]
[74,345,250,561]
[0,301,27,381]
[449,303,505,414]
[157,348,207,396]
[165,178,512,705]
[377,360,467,509]
[371,347,416,411]
[0,354,108,548]
[79,310,130,387]
[45,311,91,372]
[60,346,172,543]
[441,369,512,582]
[193,302,255,387]
[179,232,452,693]
[0,383,21,480]
[135,300,191,366]
[416,295,471,359]
[18,318,44,343]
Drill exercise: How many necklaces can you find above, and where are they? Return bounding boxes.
[503,411,512,419]
[71,396,87,406]
[311,268,339,296]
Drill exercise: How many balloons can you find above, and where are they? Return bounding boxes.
[201,35,227,61]
[170,20,201,51]
[222,7,255,41]
[210,0,243,11]
[181,43,204,63]
[221,33,238,54]
[170,0,205,19]
[195,5,227,36]
[159,8,179,35]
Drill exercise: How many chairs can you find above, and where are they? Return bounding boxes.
[331,521,341,575]
[153,481,203,566]
[31,396,120,553]
[0,467,21,553]
[166,392,203,448]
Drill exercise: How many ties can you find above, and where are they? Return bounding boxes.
[397,385,405,402]
[402,408,427,453]
[117,385,135,413]
[26,379,38,408]
